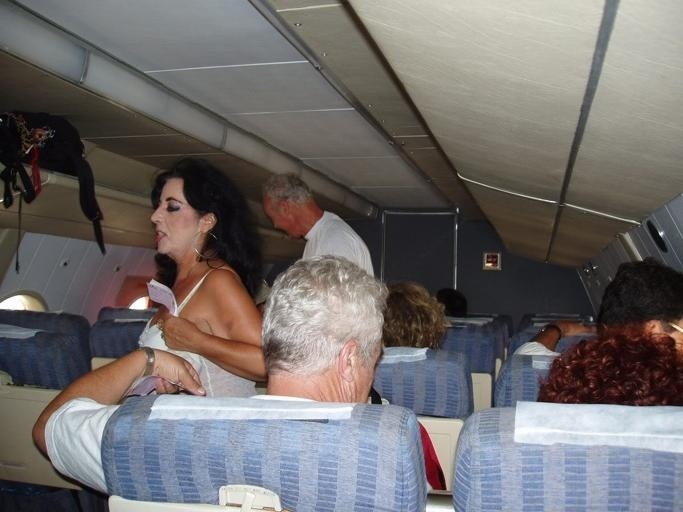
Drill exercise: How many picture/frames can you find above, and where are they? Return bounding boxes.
[481,248,503,271]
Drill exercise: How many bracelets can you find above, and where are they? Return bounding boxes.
[137,346,154,376]
[542,324,562,339]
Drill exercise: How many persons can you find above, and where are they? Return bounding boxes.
[385,280,445,350]
[437,288,467,319]
[31,256,433,496]
[264,172,376,280]
[538,327,682,406]
[138,159,266,397]
[513,257,682,356]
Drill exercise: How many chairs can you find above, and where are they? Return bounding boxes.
[0,323,105,512]
[372,346,474,496]
[493,354,559,408]
[505,328,582,357]
[96,305,160,321]
[440,325,497,407]
[452,401,683,512]
[88,319,150,371]
[0,309,92,371]
[99,394,428,512]
